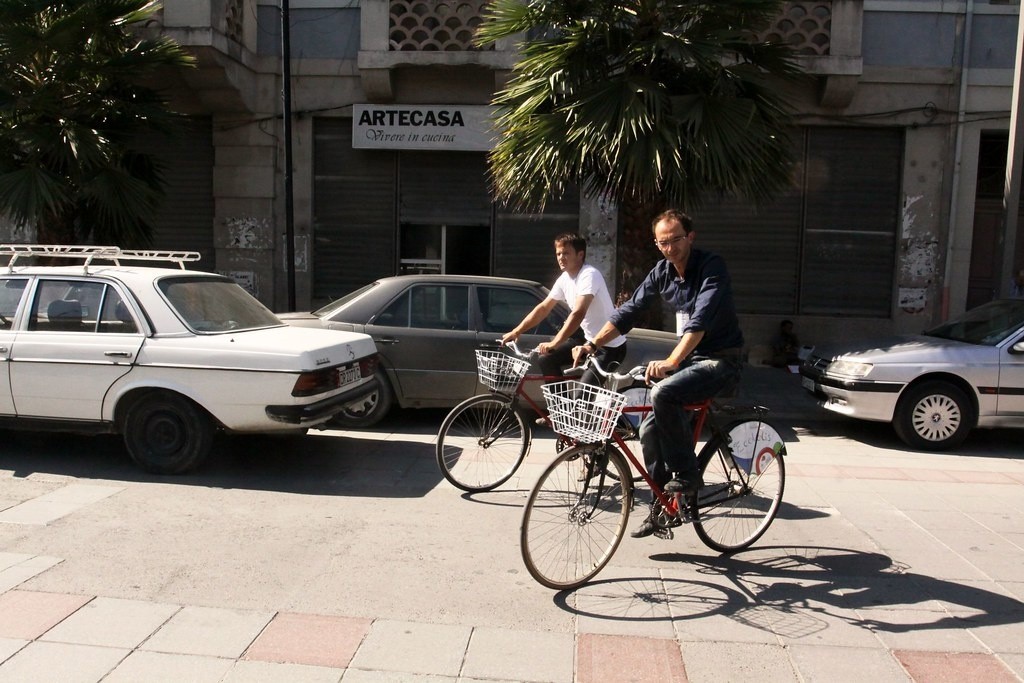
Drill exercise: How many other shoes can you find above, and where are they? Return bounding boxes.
[577,464,602,482]
[535,413,557,429]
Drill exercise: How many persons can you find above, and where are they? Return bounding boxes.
[501,233,628,480]
[570,210,746,539]
[1008,268,1024,296]
[772,320,800,369]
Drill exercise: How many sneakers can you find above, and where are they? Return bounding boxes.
[663,474,704,491]
[632,512,675,538]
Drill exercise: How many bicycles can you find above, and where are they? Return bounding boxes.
[518,352,787,591]
[437,338,661,494]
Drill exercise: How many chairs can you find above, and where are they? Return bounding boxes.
[449,301,495,332]
[115,300,134,322]
[48,299,82,331]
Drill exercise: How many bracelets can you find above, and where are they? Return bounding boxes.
[667,357,679,367]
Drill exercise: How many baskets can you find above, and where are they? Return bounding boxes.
[540,380,627,445]
[475,349,532,394]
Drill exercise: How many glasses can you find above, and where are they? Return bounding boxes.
[655,233,689,251]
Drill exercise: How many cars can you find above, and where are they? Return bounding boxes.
[270,276,739,430]
[783,295,1023,453]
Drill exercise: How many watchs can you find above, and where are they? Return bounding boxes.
[583,341,597,351]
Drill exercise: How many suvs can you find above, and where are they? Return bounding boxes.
[0,242,378,477]
